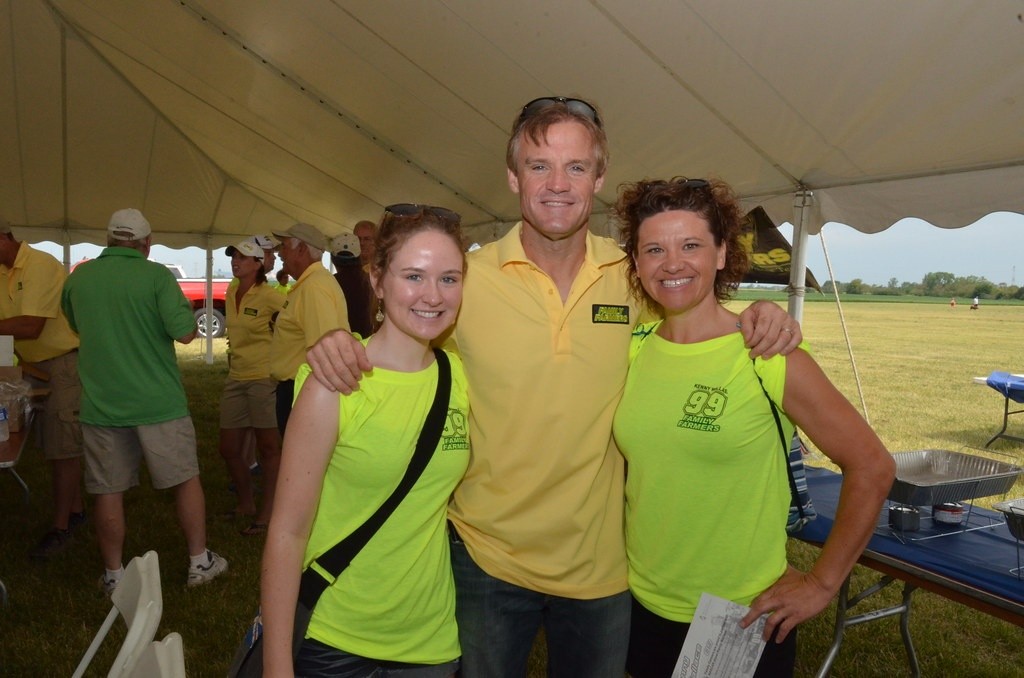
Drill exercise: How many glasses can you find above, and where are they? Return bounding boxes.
[640,175,726,237]
[378,204,461,244]
[519,96,602,130]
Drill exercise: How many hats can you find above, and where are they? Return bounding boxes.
[108,208,152,241]
[271,223,326,252]
[330,231,361,258]
[249,235,273,249]
[225,241,264,265]
[0,217,11,233]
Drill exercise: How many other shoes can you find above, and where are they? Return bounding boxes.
[30,528,74,560]
[70,509,86,526]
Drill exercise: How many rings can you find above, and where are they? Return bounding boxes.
[779,328,794,336]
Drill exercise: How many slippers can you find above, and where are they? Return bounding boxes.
[224,510,255,522]
[240,520,268,536]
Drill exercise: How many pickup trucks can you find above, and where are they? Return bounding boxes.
[71,258,234,338]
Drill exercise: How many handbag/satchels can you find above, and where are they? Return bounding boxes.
[225,566,329,678]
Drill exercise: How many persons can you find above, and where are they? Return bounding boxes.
[353,220,379,274]
[607,174,898,678]
[225,233,282,471]
[304,95,803,678]
[0,217,87,546]
[260,204,472,678]
[60,207,228,598]
[950,297,956,307]
[973,296,978,307]
[330,232,372,339]
[218,241,287,536]
[270,223,352,439]
[274,270,292,297]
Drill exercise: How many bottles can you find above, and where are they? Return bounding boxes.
[0,396,9,442]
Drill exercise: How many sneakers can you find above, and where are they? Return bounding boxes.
[187,549,228,588]
[98,574,119,602]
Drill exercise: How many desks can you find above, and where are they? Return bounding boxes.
[785,464,1024,677]
[0,409,38,495]
[973,374,1024,446]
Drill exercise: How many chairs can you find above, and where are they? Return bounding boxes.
[71,549,188,678]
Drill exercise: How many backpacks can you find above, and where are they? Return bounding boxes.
[752,343,818,535]
